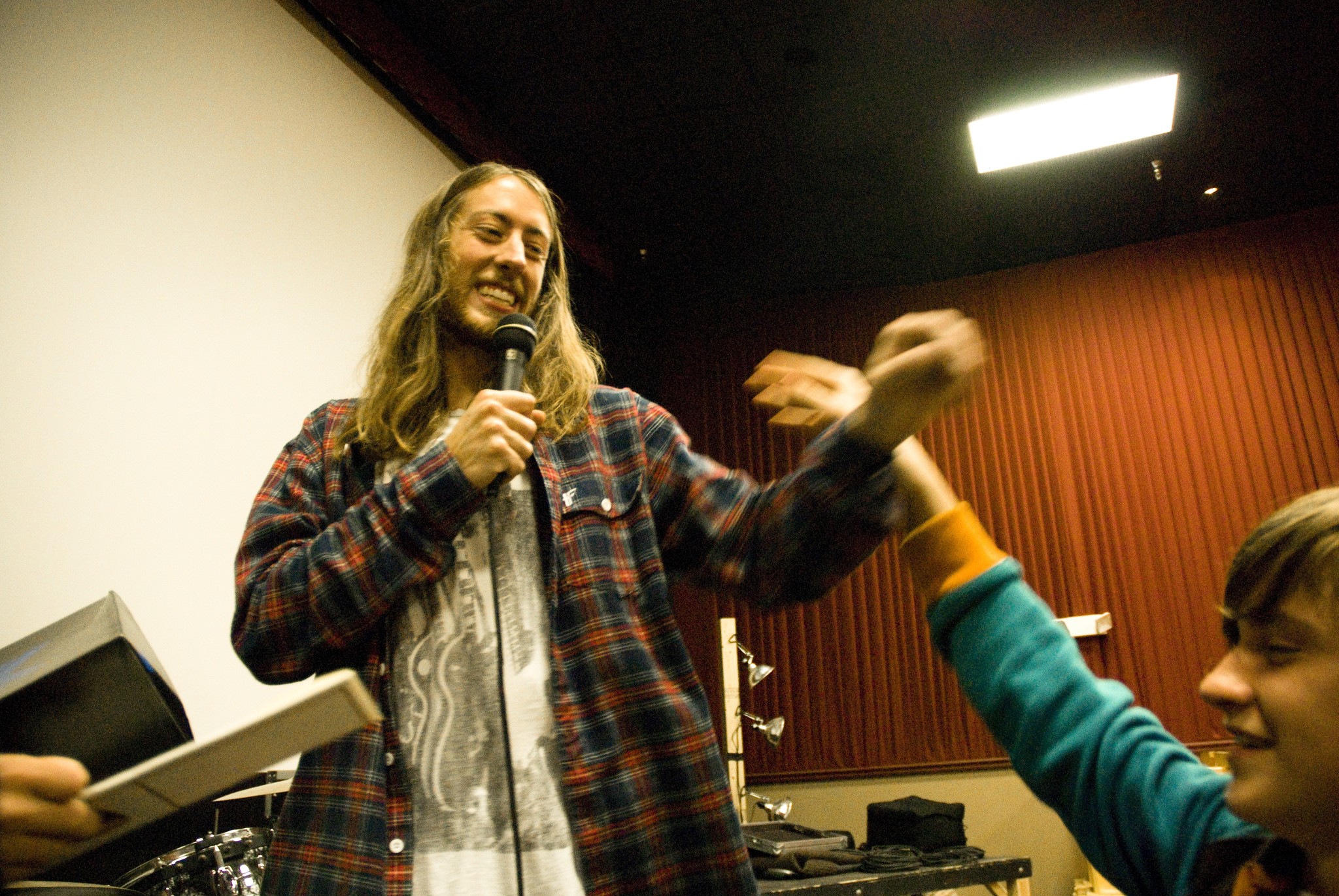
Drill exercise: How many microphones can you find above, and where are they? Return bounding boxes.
[488,313,538,497]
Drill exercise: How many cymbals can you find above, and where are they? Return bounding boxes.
[212,776,294,802]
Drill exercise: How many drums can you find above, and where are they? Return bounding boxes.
[113,825,278,896]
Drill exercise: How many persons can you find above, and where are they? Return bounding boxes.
[227,157,987,896]
[0,753,104,882]
[741,348,1339,896]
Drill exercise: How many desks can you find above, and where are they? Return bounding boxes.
[757,854,1032,896]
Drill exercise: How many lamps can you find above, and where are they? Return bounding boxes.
[743,788,793,821]
[741,711,786,746]
[737,644,775,690]
